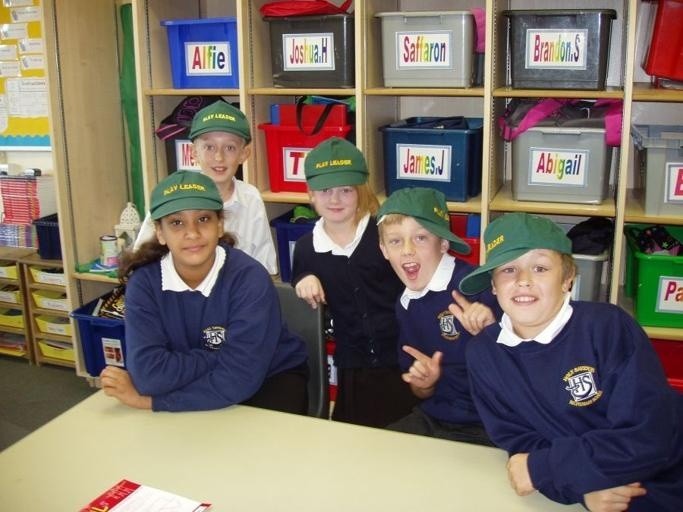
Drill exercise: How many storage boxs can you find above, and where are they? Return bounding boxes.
[624,225,683,328]
[29,266,66,285]
[569,250,612,304]
[31,292,71,309]
[160,16,239,90]
[173,137,200,176]
[34,316,69,333]
[372,8,474,90]
[507,125,614,204]
[500,8,617,90]
[70,287,136,378]
[0,313,25,327]
[38,340,73,359]
[630,124,682,216]
[269,206,315,282]
[263,13,353,90]
[0,290,21,302]
[258,122,352,192]
[30,212,61,261]
[0,264,18,279]
[378,115,484,203]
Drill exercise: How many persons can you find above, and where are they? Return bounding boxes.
[375,186,504,450]
[458,212,683,512]
[133,100,280,276]
[98,169,310,416]
[290,136,406,430]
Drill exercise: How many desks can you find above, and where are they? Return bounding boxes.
[1,385,583,512]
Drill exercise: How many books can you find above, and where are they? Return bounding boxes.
[0,175,57,250]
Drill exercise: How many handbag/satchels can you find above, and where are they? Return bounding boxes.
[294,95,352,136]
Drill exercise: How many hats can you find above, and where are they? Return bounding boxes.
[375,186,472,256]
[187,99,252,143]
[303,134,370,191]
[458,212,573,296]
[149,168,224,221]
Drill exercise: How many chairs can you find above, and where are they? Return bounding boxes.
[272,284,324,421]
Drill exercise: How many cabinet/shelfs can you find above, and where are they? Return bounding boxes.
[0,244,35,365]
[127,0,683,342]
[18,255,78,371]
